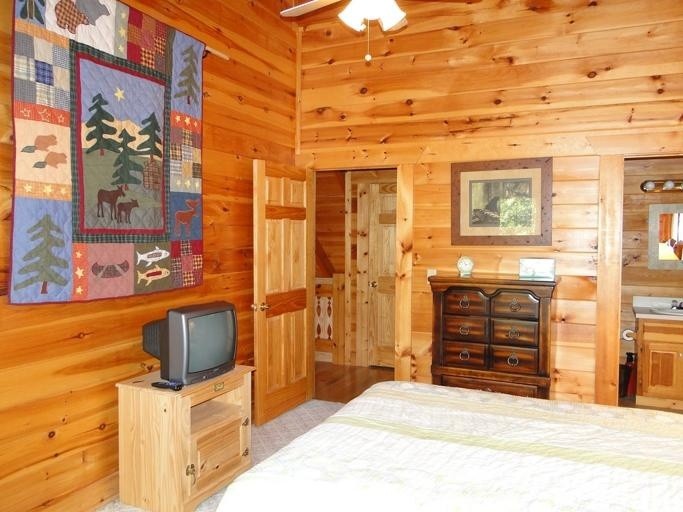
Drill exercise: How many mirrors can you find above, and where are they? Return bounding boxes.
[647,202,683,271]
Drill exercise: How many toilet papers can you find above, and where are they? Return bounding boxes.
[623,329,635,341]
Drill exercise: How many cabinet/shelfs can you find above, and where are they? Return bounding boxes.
[114,362,256,512]
[428,274,555,400]
[631,318,683,411]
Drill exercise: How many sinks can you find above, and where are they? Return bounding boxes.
[651,307,683,315]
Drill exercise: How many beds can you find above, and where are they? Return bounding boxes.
[216,379,683,512]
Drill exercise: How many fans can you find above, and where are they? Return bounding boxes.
[277,0,344,20]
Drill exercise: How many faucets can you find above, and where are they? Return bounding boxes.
[679,302,683,307]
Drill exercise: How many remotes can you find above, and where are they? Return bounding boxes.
[151,382,182,391]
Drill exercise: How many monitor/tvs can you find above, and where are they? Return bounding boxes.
[141,301,238,387]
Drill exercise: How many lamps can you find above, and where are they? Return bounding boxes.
[337,0,409,62]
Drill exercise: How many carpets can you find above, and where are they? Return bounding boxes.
[89,390,347,512]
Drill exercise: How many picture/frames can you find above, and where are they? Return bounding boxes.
[449,157,553,246]
